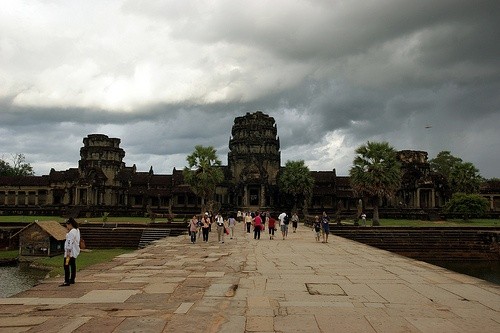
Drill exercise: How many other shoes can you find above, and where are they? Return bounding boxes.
[64,279,75,284]
[59,283,70,287]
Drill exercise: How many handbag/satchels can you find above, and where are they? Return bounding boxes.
[224,227,229,235]
[260,225,264,230]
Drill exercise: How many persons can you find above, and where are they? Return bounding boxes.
[59,217,80,286]
[252,211,262,240]
[242,209,270,233]
[278,209,288,240]
[268,214,275,240]
[227,215,236,239]
[361,213,366,227]
[321,212,330,243]
[312,216,322,241]
[201,212,212,242]
[237,209,241,223]
[291,212,298,233]
[187,215,199,243]
[216,214,225,243]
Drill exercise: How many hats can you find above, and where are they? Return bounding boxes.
[205,212,208,216]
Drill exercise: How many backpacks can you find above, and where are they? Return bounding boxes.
[314,220,320,232]
[283,213,289,225]
